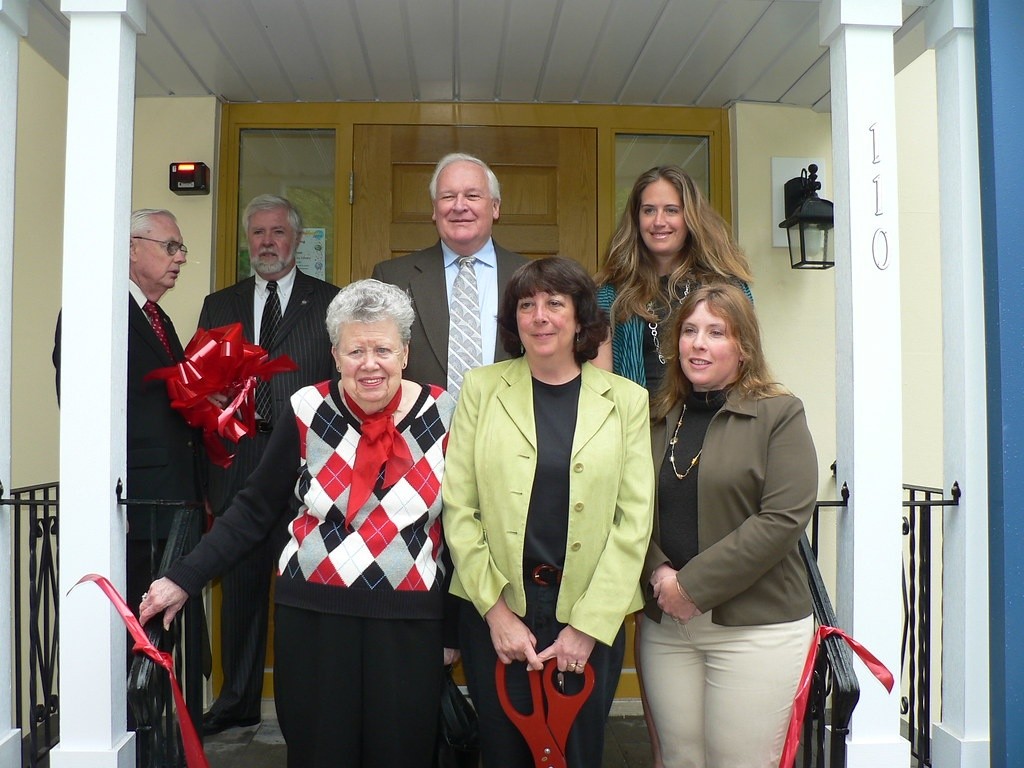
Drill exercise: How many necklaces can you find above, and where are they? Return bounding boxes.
[669,404,702,479]
[647,280,690,364]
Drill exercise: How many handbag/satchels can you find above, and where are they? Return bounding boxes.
[438,659,481,768]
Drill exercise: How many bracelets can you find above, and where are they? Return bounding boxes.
[676,580,693,603]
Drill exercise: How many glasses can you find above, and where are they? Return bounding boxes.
[133,235,188,258]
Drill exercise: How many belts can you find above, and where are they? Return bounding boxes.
[252,419,274,434]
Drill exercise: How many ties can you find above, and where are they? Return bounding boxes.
[145,300,175,364]
[254,278,282,424]
[446,255,482,403]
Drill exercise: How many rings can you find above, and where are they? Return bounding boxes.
[577,663,584,668]
[142,592,147,601]
[568,663,576,667]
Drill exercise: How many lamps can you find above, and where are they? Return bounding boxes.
[771,158,833,269]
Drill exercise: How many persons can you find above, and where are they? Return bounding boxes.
[445,256,655,768]
[640,283,819,768]
[588,165,754,768]
[370,153,532,401]
[197,194,342,737]
[139,278,460,768]
[52,209,228,768]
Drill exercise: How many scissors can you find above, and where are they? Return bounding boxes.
[495,656,594,768]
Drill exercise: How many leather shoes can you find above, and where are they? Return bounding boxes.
[202,710,262,734]
